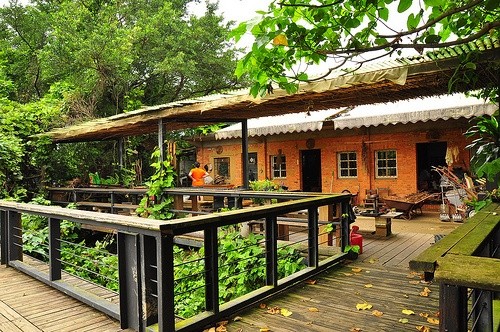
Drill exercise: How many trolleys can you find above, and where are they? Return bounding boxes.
[382,193,442,220]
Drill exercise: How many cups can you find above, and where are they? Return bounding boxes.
[390,208,396,215]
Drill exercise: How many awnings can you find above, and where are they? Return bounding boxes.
[332,87,500,131]
[213,106,348,141]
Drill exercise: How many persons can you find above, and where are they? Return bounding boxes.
[189,162,210,203]
[203,165,212,184]
